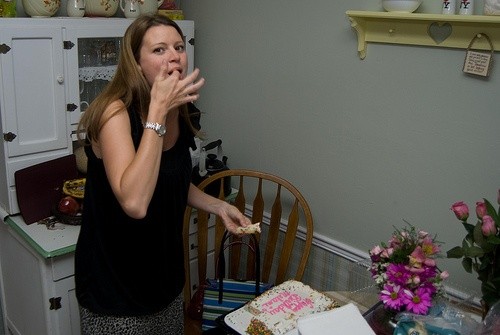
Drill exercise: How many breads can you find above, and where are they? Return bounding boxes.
[237,222,261,233]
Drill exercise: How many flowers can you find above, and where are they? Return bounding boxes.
[445,189,500,322]
[370,217,449,315]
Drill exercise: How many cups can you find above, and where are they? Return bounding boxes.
[120,0,141,18]
[67,0,86,17]
[459,0,474,14]
[141,0,164,17]
[441,0,455,14]
[0,0,17,17]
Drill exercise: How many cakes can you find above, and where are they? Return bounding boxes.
[246,280,339,335]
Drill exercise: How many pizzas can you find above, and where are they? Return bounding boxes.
[63,178,85,198]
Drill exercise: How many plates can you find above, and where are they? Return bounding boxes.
[54,208,85,225]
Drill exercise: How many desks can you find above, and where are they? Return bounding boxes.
[323,290,482,335]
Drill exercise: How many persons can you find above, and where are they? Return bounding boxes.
[74,14,251,335]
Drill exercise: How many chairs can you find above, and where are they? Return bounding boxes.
[184,169,314,335]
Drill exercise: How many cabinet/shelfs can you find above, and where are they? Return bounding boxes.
[0,16,239,335]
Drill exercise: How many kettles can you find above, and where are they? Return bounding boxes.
[192,139,232,198]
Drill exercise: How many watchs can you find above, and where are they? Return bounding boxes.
[144,122,167,137]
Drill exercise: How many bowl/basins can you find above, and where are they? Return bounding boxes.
[382,0,421,13]
[23,0,62,18]
[86,0,119,17]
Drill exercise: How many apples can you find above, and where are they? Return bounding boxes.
[58,197,79,214]
